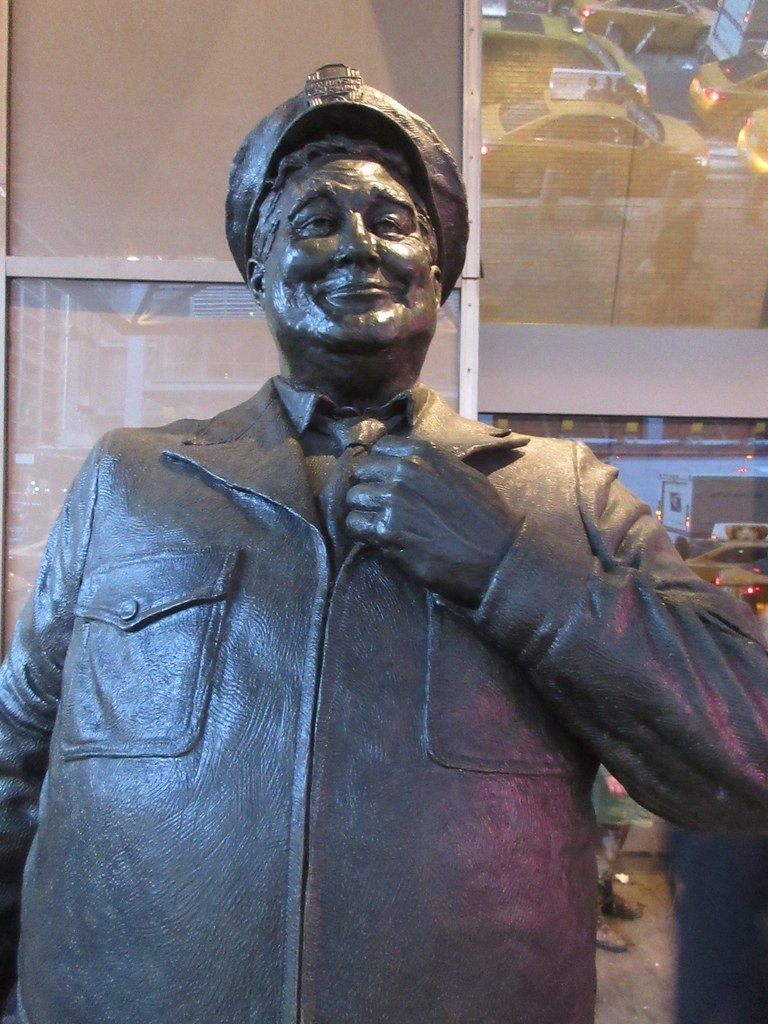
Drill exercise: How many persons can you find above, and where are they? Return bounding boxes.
[0,64,767,1024]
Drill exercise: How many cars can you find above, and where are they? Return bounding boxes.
[740,108,767,186]
[483,86,708,198]
[575,0,717,48]
[686,529,767,584]
[688,53,767,129]
[480,8,648,100]
